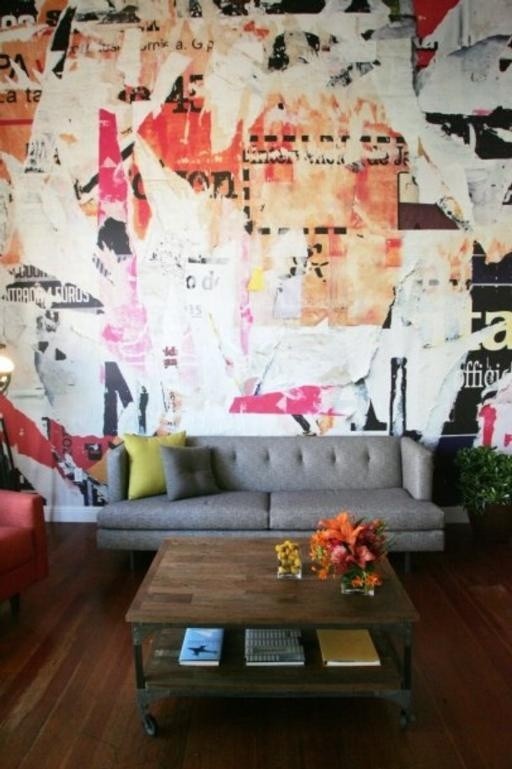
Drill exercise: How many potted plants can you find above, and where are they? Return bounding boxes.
[460,446,512,543]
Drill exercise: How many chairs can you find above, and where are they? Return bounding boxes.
[0,488,50,612]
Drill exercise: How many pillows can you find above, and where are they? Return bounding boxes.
[121,432,188,501]
[160,442,221,502]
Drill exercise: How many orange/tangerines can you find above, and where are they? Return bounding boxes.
[275,540,303,576]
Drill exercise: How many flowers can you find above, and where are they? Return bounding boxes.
[310,511,389,590]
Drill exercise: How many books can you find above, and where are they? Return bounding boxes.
[177,627,225,667]
[315,628,382,668]
[246,628,307,666]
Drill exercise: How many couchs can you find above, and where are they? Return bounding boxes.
[96,434,444,574]
